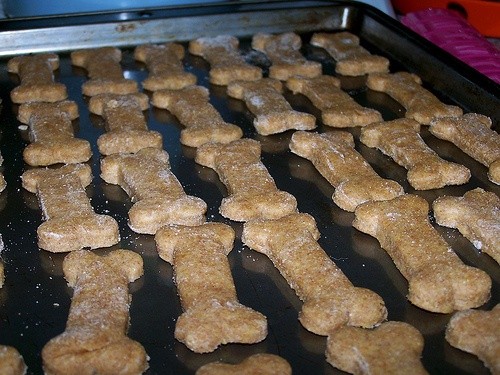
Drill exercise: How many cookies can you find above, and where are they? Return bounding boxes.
[0,31,500,375]
